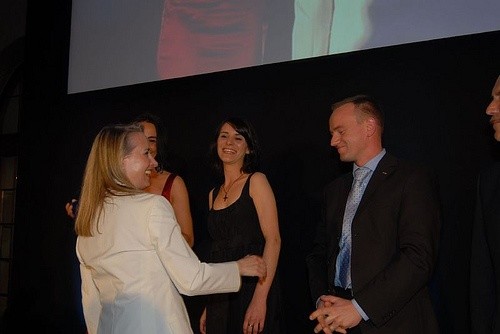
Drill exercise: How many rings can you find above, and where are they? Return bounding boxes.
[248,324,254,328]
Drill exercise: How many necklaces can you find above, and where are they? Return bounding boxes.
[222,173,246,201]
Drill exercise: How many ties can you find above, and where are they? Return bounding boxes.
[335,168,372,291]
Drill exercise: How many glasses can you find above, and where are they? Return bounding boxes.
[148,136,160,145]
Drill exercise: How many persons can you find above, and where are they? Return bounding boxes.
[200,116,281,334]
[65,115,194,249]
[309,93,431,334]
[291,0,373,60]
[467,74,500,334]
[156,0,266,79]
[74,123,267,334]
